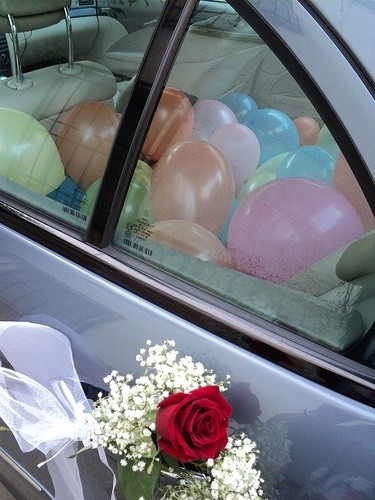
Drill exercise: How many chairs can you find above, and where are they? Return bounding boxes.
[103,25,269,101]
[0,0,120,141]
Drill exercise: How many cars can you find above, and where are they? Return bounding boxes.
[2,0,375,500]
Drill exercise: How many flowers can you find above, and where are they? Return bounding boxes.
[1,336,265,500]
